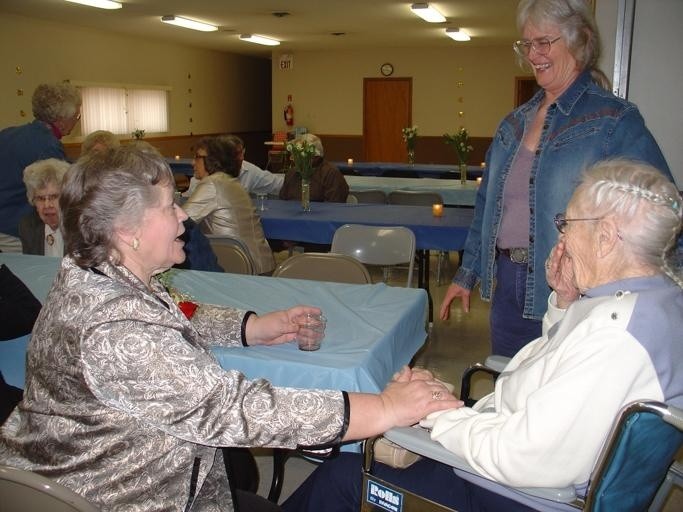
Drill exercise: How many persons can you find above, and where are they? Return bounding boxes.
[180,136,277,277]
[279,158,683,512]
[269,134,350,253]
[1,82,84,240]
[0,142,465,512]
[439,0,683,388]
[74,124,119,162]
[16,154,77,260]
[181,135,286,197]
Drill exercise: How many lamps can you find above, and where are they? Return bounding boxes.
[162,15,218,32]
[411,3,446,23]
[446,27,471,42]
[239,34,280,46]
[65,0,122,10]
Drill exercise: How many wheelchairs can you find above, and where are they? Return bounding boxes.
[358,352,683,511]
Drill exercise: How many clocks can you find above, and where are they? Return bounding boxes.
[381,63,394,76]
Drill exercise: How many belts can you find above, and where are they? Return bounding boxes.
[502,248,529,264]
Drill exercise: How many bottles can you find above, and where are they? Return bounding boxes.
[302,179,310,212]
[408,148,415,167]
[461,164,466,184]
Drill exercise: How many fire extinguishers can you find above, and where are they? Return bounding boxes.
[284,102,293,126]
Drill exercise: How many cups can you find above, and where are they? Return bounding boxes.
[256,193,268,212]
[296,314,327,351]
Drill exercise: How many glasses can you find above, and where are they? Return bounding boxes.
[34,193,59,202]
[238,147,246,154]
[513,36,561,57]
[192,152,205,160]
[553,213,599,234]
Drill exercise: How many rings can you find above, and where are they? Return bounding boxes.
[546,265,551,269]
[431,391,441,401]
